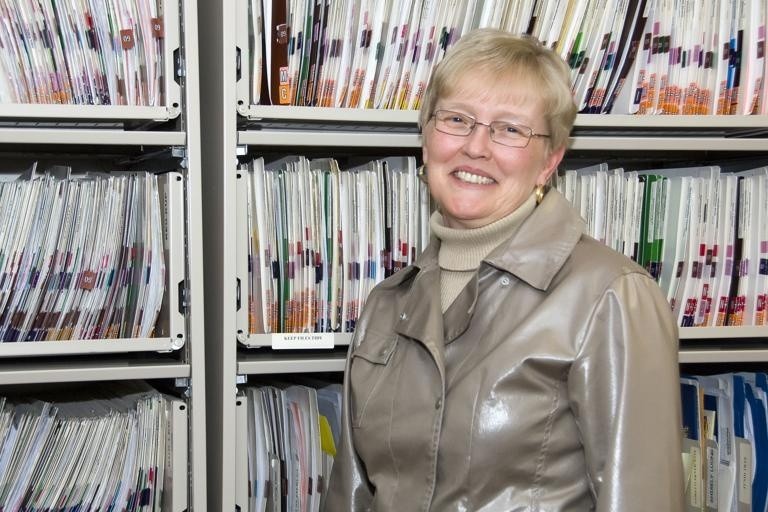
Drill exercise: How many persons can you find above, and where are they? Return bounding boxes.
[322,30,688,511]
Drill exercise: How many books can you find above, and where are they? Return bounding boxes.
[678,369,768,512]
[250,388,345,510]
[235,155,767,336]
[1,170,166,341]
[238,2,765,114]
[2,1,162,105]
[0,387,171,512]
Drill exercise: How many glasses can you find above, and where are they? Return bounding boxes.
[424,107,555,148]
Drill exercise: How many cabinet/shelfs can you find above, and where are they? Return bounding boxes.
[0,0,199,511]
[222,0,768,510]
